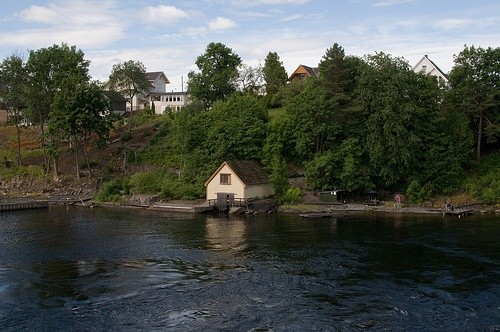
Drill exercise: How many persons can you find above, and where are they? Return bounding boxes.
[446,201,455,211]
